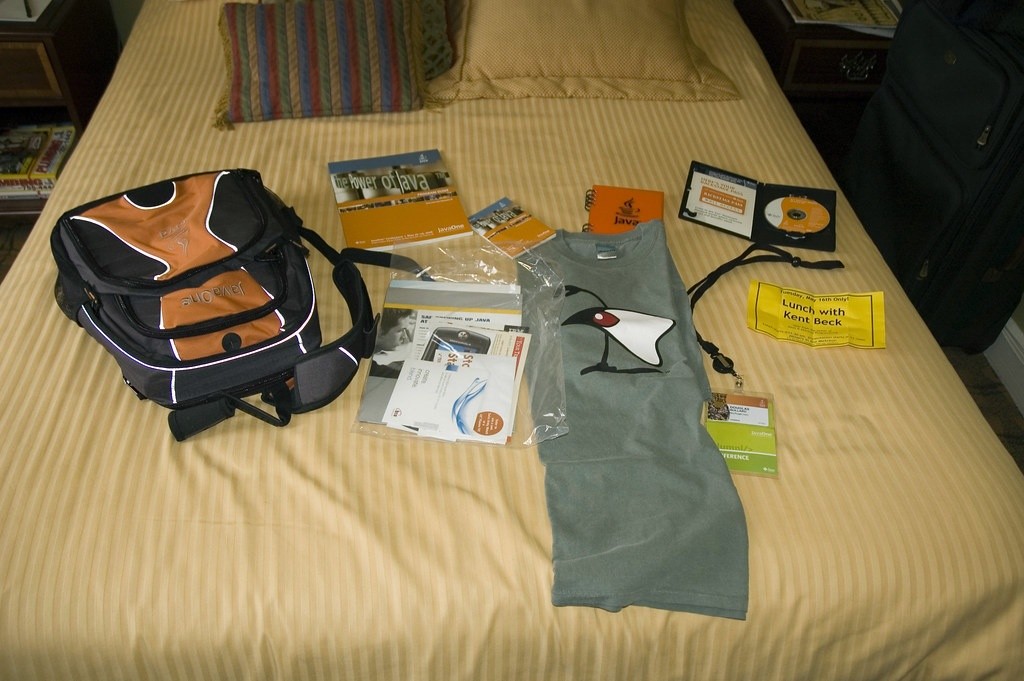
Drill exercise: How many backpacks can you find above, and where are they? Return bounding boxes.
[836,0,1023,354]
[50,170,433,441]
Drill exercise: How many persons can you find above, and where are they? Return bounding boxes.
[370,308,417,378]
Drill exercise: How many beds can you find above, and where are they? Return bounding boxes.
[0,0,1024,681]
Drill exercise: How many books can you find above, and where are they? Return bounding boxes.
[582,185,666,235]
[328,147,473,250]
[468,196,557,258]
[0,123,76,202]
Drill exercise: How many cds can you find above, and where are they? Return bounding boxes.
[764,196,830,235]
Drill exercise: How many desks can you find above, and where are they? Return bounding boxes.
[0,0,125,226]
[749,0,893,170]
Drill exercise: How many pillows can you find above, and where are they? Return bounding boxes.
[215,0,739,126]
